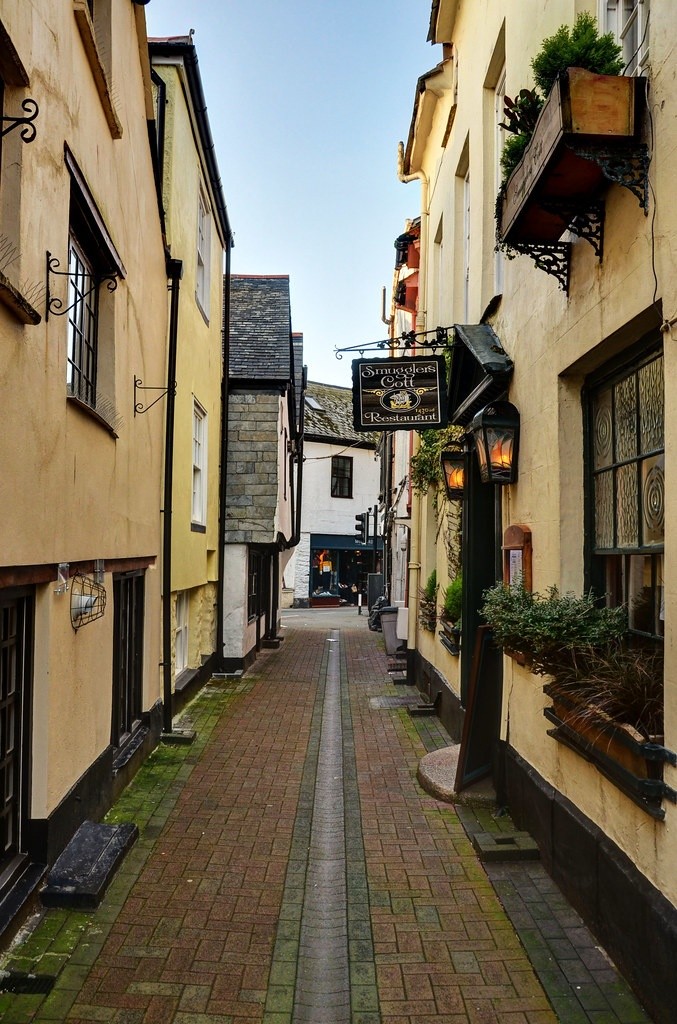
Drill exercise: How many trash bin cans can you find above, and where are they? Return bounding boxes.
[378,606,407,656]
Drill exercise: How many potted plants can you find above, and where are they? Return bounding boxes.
[491,9,648,260]
[436,572,464,644]
[476,569,633,681]
[417,569,436,621]
[539,674,664,782]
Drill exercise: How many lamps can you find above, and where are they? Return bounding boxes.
[466,400,520,485]
[440,441,469,501]
